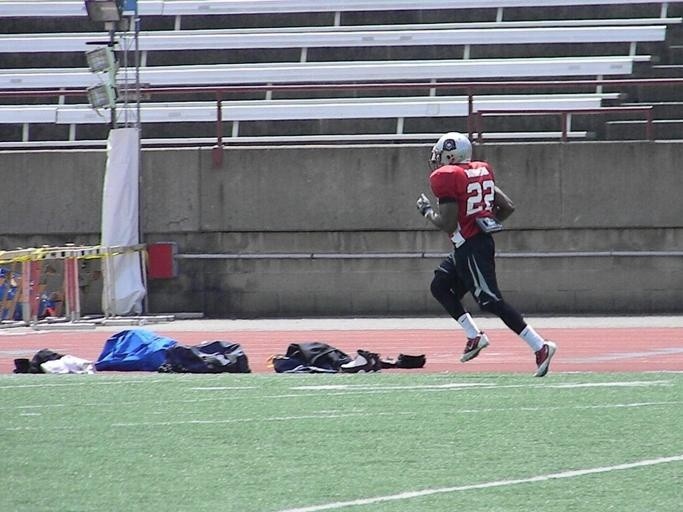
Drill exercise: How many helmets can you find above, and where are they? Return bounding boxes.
[430,131,473,165]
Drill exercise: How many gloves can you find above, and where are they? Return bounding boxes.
[416,193,432,216]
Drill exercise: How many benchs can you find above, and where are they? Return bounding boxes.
[0,0,683,148]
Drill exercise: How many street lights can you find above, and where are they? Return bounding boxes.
[85,0,125,126]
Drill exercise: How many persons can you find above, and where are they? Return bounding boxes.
[416,131,557,376]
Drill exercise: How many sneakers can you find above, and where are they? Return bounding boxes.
[459,331,490,362]
[532,341,557,377]
